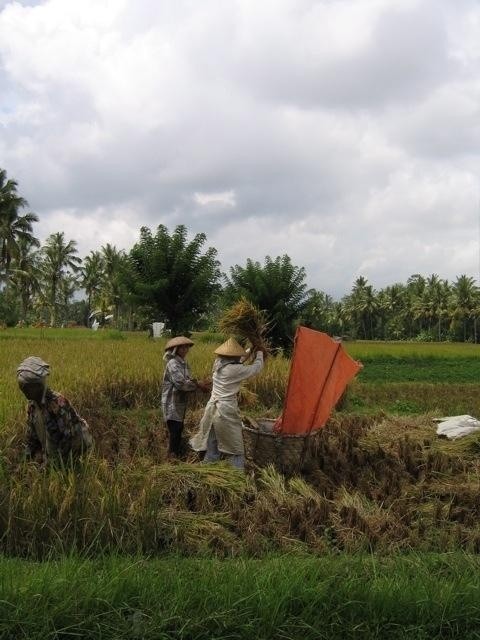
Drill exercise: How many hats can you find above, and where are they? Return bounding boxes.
[213,336,249,357]
[15,355,50,385]
[165,336,194,352]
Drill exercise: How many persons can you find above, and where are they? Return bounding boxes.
[15,355,97,475]
[160,335,210,465]
[189,337,265,470]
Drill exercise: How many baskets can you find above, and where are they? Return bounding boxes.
[240,417,321,475]
